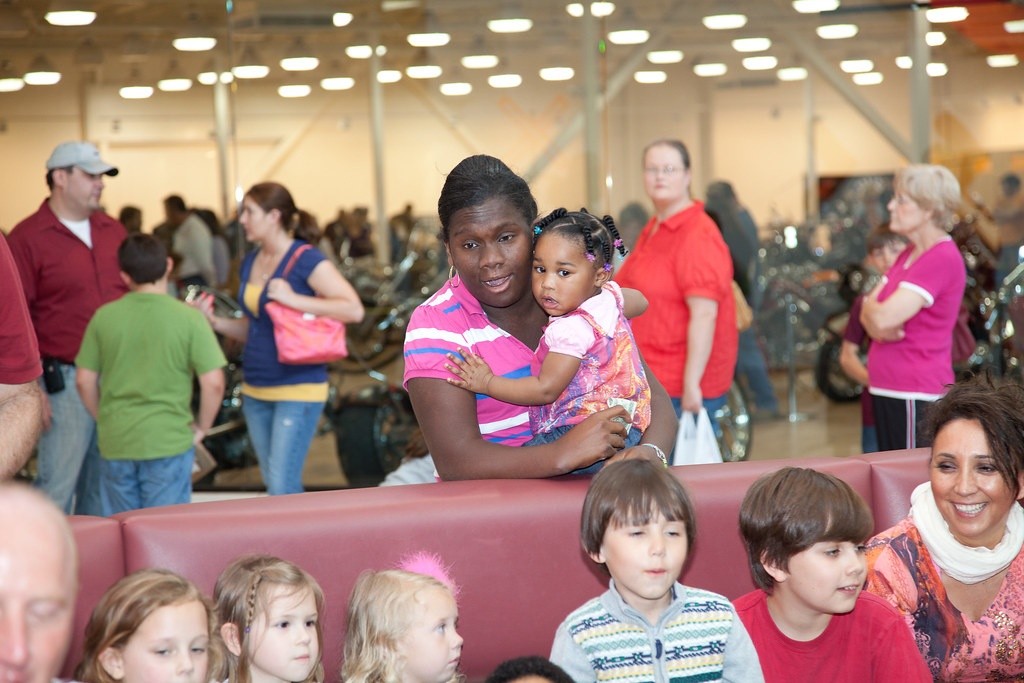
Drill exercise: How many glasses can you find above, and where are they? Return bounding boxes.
[643,166,685,175]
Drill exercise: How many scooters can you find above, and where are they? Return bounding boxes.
[755,215,1024,412]
[165,199,451,489]
[713,375,752,463]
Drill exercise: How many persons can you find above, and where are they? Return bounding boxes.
[0,234,42,478]
[76,232,227,516]
[732,465,934,683]
[613,143,739,445]
[403,155,680,481]
[212,554,325,683]
[980,175,1024,289]
[322,205,415,266]
[707,178,783,418]
[189,181,365,496]
[840,168,974,450]
[340,557,465,683]
[548,459,766,683]
[0,480,79,683]
[617,200,649,248]
[862,380,1024,683]
[443,208,651,477]
[76,568,226,683]
[121,194,230,294]
[486,656,574,683]
[9,140,128,513]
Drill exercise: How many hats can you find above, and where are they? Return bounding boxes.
[48,142,118,177]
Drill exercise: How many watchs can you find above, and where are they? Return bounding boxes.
[642,443,667,469]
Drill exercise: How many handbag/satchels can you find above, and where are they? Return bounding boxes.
[264,246,348,365]
[672,409,724,467]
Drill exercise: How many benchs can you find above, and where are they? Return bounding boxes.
[58,447,929,683]
